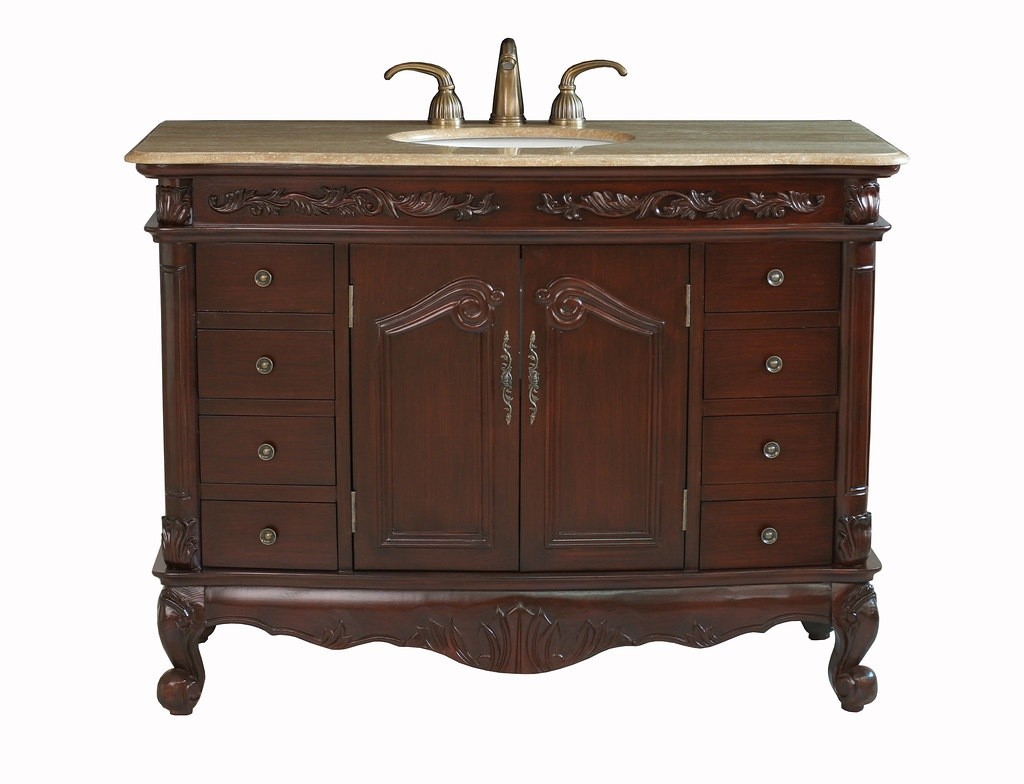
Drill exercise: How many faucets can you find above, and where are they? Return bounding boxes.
[488,38,527,124]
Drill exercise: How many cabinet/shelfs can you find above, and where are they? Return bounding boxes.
[134,162,892,713]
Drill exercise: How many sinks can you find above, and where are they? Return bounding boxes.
[387,129,635,148]
